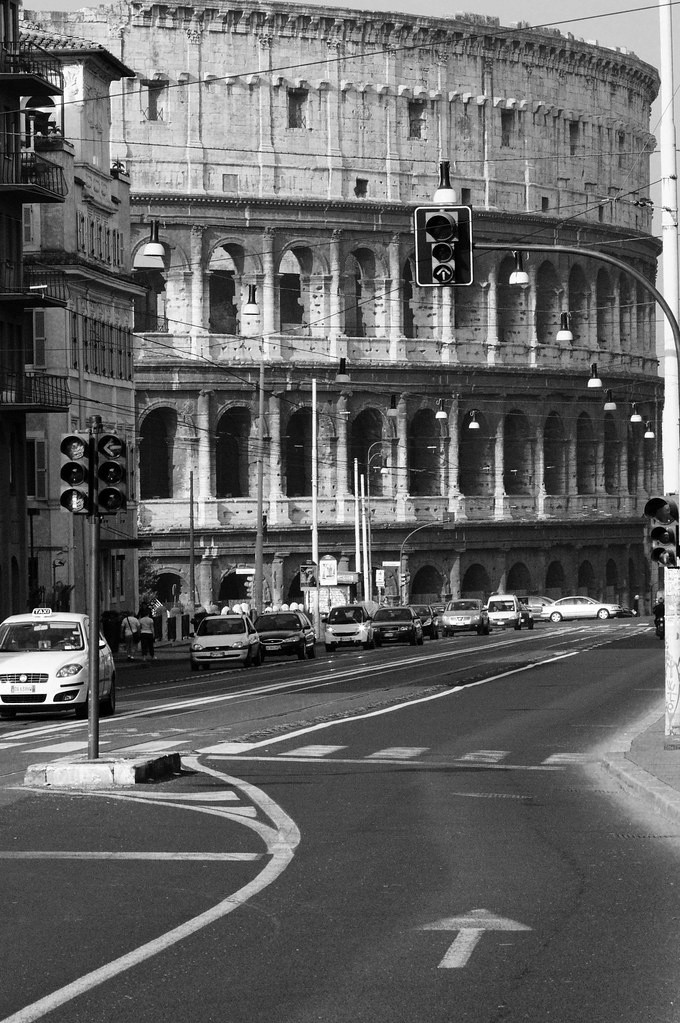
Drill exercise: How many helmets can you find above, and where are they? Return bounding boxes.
[658,598,664,603]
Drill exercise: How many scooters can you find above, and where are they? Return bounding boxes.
[653,617,665,640]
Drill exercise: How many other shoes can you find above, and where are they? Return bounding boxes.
[128,657,134,661]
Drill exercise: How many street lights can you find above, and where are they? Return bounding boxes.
[398,519,452,607]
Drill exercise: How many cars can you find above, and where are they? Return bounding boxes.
[187,613,260,672]
[406,604,439,640]
[540,595,624,623]
[429,601,449,632]
[371,605,424,647]
[483,594,534,631]
[322,604,375,652]
[252,609,316,663]
[516,595,559,622]
[0,607,117,721]
[440,598,491,638]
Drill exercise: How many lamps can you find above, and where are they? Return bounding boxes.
[435,400,447,419]
[387,396,400,417]
[587,365,602,387]
[468,414,480,429]
[509,252,528,284]
[556,313,573,342]
[644,423,655,438]
[433,160,456,204]
[242,285,260,315]
[604,389,617,410]
[630,407,642,422]
[335,359,350,383]
[144,220,165,257]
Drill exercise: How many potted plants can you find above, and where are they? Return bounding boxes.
[35,117,63,150]
[110,156,126,180]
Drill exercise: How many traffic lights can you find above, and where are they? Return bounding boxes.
[58,432,90,514]
[643,493,680,569]
[400,573,406,588]
[414,206,474,287]
[96,432,128,513]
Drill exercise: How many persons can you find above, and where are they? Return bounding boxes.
[121,609,140,660]
[305,568,316,587]
[653,597,665,630]
[139,610,157,662]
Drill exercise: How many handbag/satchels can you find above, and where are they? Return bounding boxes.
[133,632,141,643]
